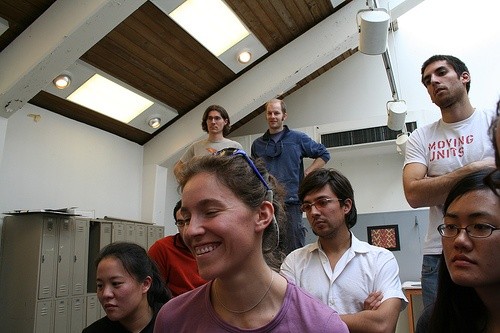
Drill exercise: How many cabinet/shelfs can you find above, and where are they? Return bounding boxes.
[0,213,165,333]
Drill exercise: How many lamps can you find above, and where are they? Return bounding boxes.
[396,132,411,157]
[386,91,407,131]
[355,0,390,57]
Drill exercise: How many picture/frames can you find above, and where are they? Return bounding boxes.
[367,224,400,252]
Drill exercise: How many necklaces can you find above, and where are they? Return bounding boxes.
[214,269,274,314]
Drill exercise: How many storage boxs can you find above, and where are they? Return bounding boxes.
[67,208,95,219]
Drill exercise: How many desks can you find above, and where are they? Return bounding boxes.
[401,280,423,333]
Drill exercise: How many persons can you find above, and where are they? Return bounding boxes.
[403,55,500,333]
[148,200,209,298]
[175,105,243,170]
[251,99,331,261]
[82,241,160,333]
[427,167,500,333]
[279,168,408,333]
[154,148,349,333]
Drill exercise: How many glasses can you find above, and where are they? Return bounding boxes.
[301,198,342,213]
[213,147,269,190]
[175,220,185,227]
[437,223,500,238]
